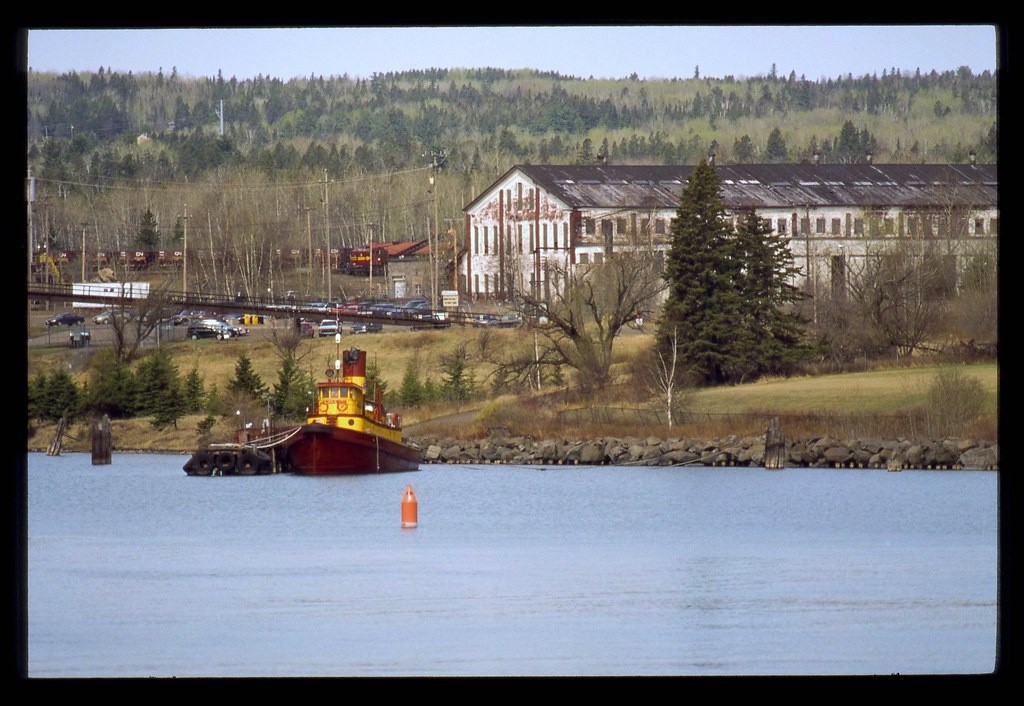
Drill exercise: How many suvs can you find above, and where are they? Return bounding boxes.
[184,318,234,339]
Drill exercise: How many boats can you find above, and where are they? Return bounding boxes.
[283,298,424,480]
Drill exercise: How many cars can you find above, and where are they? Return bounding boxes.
[472,315,498,326]
[70,328,91,342]
[92,310,131,326]
[349,321,382,335]
[493,314,521,327]
[45,311,87,326]
[134,306,265,325]
[300,321,315,338]
[317,318,344,337]
[307,299,433,320]
[220,321,249,335]
